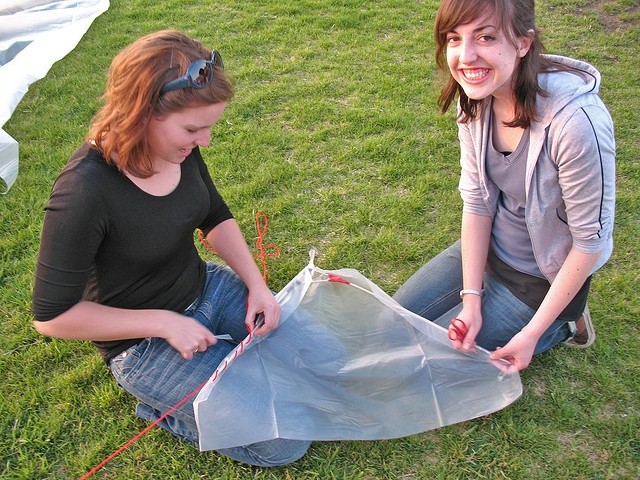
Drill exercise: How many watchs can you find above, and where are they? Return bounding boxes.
[460,289,481,298]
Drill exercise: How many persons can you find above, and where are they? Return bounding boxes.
[31,29,355,468]
[377,2,616,375]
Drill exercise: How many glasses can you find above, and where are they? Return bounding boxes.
[160,50,224,94]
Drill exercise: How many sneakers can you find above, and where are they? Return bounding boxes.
[564,305,596,350]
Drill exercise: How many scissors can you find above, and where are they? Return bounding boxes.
[447,318,480,355]
